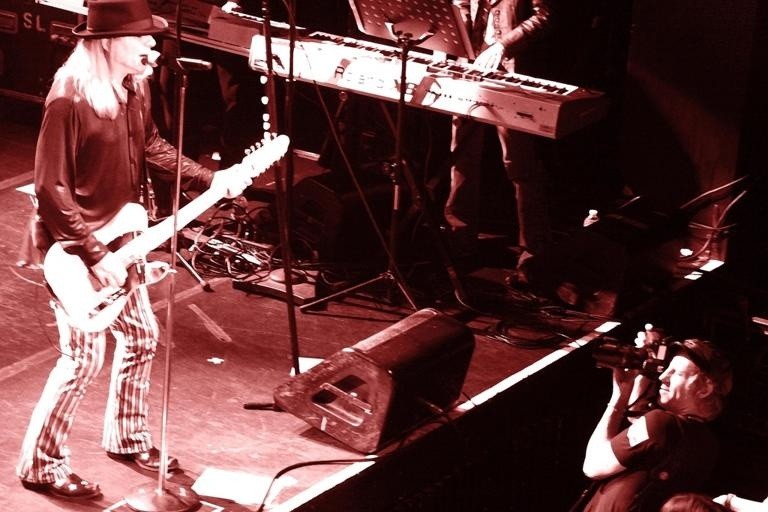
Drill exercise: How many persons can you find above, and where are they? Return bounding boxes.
[447,0,553,275]
[21,0,253,501]
[571,322,733,512]
[660,492,732,512]
[157,0,255,209]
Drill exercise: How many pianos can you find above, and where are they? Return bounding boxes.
[249,30,611,140]
[148,0,307,63]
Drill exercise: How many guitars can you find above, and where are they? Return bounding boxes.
[44,131,291,333]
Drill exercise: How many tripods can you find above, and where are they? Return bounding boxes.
[298,18,436,314]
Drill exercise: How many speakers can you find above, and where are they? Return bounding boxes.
[273,307,477,455]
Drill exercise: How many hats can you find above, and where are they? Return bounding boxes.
[669,335,736,399]
[70,0,170,40]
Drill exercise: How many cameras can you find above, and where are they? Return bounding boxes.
[591,328,666,381]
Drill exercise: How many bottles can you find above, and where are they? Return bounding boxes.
[582,209,600,229]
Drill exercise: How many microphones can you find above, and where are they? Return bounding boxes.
[146,50,212,72]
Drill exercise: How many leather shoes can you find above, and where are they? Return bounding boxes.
[107,446,179,472]
[17,467,101,501]
